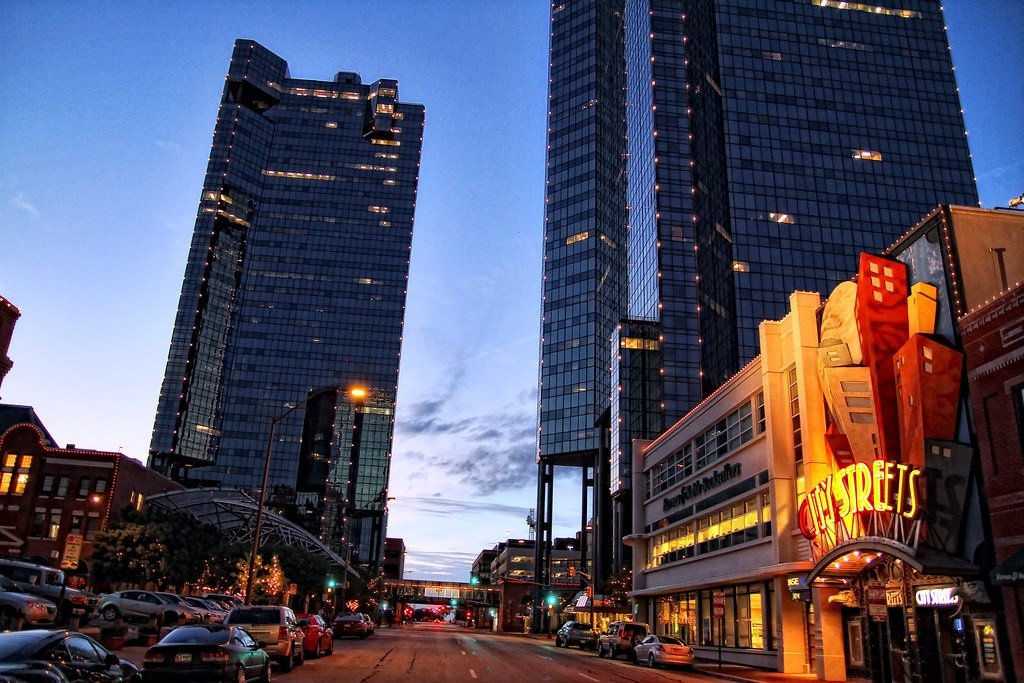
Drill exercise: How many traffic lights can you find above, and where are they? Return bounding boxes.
[452,599,457,606]
[547,595,558,609]
[382,604,387,611]
[327,580,335,595]
[470,575,479,586]
[490,610,497,615]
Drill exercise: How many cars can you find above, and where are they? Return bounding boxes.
[632,635,695,671]
[0,575,57,631]
[295,614,334,657]
[0,629,142,683]
[143,624,271,683]
[98,593,253,626]
[332,612,374,639]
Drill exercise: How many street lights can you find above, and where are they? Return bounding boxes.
[244,387,365,606]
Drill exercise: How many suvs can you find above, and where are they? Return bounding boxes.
[598,621,651,660]
[556,621,597,651]
[223,606,307,673]
[0,556,100,628]
